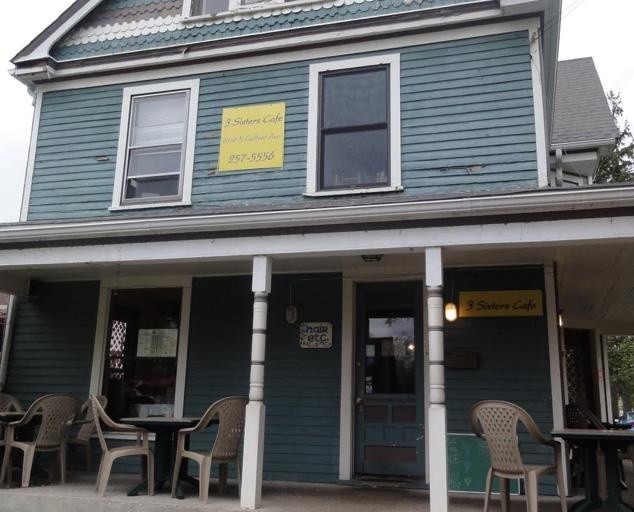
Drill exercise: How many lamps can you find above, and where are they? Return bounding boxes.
[285,279,298,323]
[444,271,458,322]
[406,317,415,351]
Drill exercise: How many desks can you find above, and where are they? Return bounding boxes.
[121,415,219,499]
[0,411,43,487]
[551,426,634,511]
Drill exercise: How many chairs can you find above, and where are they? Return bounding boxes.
[5,391,80,488]
[138,404,175,420]
[68,395,108,472]
[467,399,562,512]
[564,403,632,489]
[87,393,157,497]
[0,391,22,412]
[171,395,248,505]
